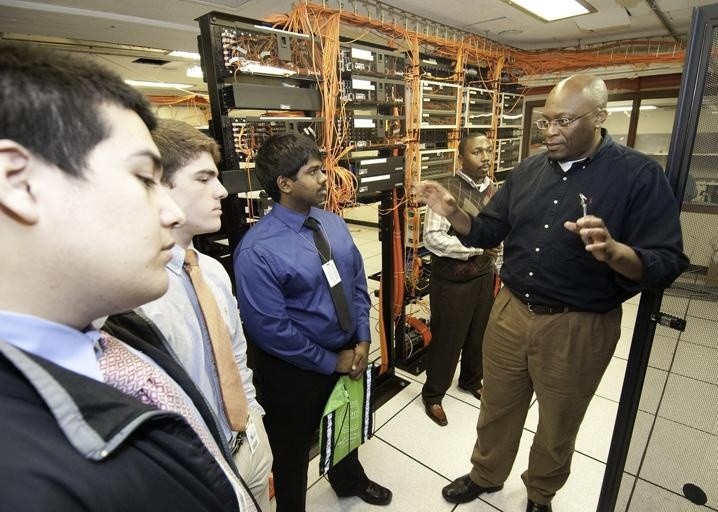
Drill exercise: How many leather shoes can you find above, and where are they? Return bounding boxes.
[458,379,483,399]
[442,474,503,503]
[527,498,552,512]
[355,475,392,506]
[426,403,448,426]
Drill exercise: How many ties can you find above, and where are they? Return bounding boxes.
[303,218,353,334]
[97,331,258,512]
[182,249,250,433]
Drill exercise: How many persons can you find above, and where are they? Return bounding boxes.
[413,73,689,511]
[1,41,259,512]
[142,116,273,512]
[421,130,506,427]
[233,133,393,511]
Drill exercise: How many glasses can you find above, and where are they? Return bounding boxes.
[536,105,602,130]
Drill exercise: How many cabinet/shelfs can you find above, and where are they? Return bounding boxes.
[516,70,716,277]
[195,9,415,469]
[388,46,528,379]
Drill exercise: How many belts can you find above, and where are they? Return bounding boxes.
[230,432,247,457]
[522,300,577,314]
